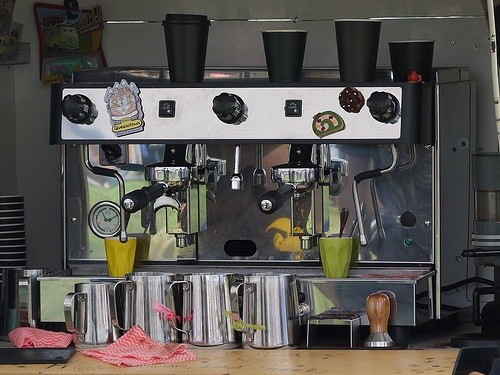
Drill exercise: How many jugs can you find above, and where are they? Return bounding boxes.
[111,271,179,344]
[164,273,235,345]
[231,273,303,351]
[64,283,113,345]
[1,268,51,342]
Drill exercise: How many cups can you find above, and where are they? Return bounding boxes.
[0,193,27,288]
[388,39,435,84]
[261,30,308,83]
[317,237,353,278]
[105,236,137,281]
[334,19,381,83]
[162,14,211,82]
